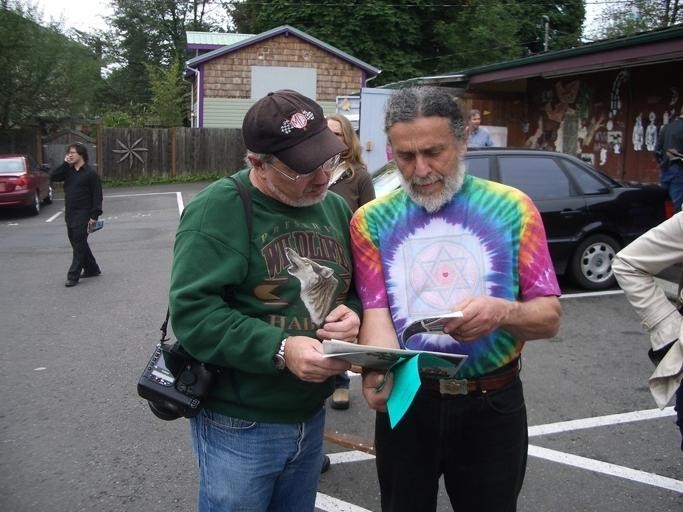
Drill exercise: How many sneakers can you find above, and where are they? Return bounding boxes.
[65,280,76,286]
[80,270,101,278]
[329,387,350,410]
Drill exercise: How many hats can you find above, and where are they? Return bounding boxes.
[243,90,348,174]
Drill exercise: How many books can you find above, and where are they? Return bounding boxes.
[320,311,469,430]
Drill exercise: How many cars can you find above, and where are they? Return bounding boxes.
[0,155,53,216]
[367,149,673,288]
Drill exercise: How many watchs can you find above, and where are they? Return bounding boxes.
[271,338,288,373]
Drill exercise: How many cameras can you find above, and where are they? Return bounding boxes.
[137,345,217,421]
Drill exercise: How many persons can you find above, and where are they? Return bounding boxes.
[346,84,566,512]
[45,143,104,288]
[607,208,683,454]
[465,108,496,148]
[168,89,361,512]
[310,111,377,413]
[654,96,683,216]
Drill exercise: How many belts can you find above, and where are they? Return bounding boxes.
[420,369,517,396]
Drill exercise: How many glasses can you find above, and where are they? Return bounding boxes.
[68,151,77,155]
[267,154,341,184]
